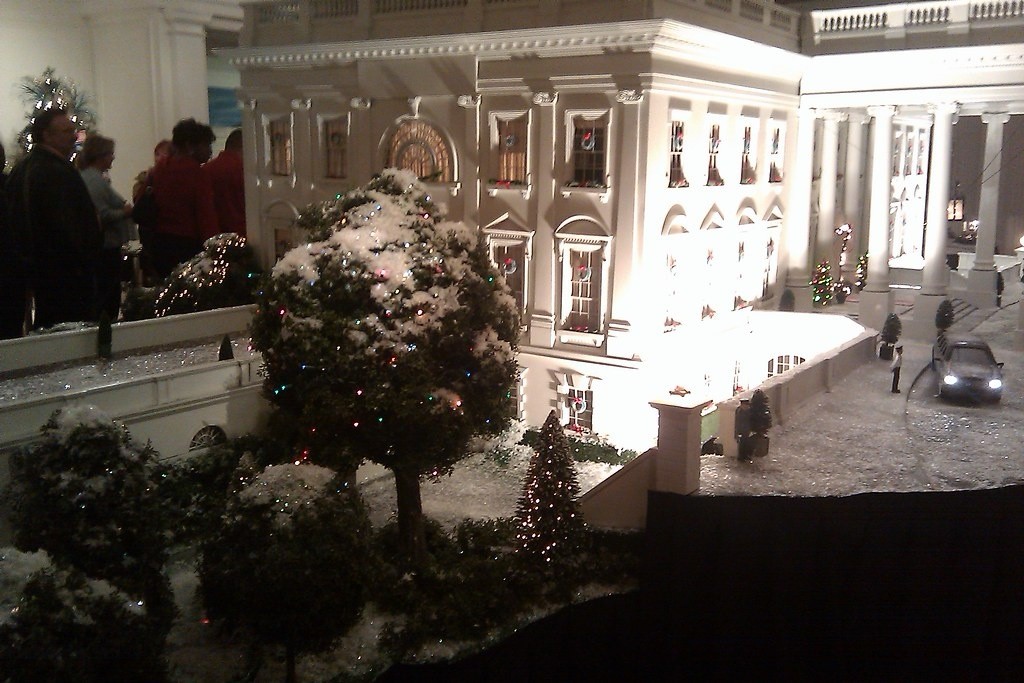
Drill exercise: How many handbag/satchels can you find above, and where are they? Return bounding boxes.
[132,174,160,225]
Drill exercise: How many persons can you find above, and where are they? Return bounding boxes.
[890,346,903,393]
[4,107,99,330]
[0,144,25,340]
[133,119,246,287]
[80,134,132,323]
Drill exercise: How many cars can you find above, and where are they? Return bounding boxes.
[931,332,1003,405]
